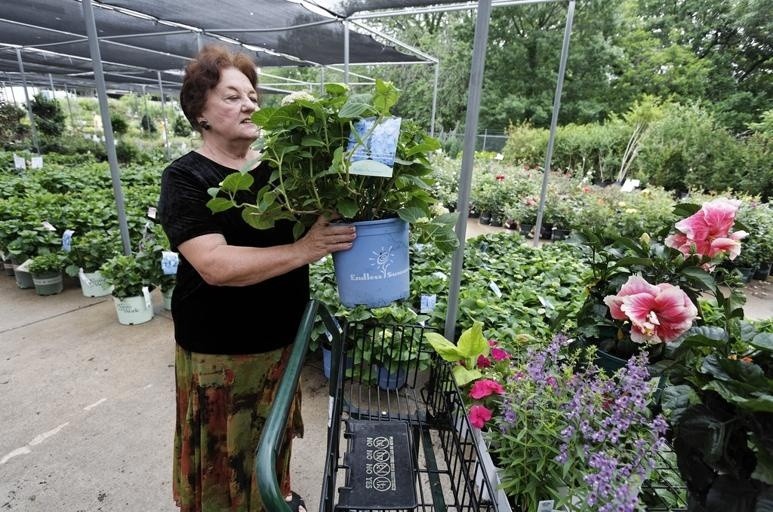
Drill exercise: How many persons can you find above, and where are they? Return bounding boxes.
[157,49,359,512]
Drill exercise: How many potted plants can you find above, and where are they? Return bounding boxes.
[0,218,181,325]
